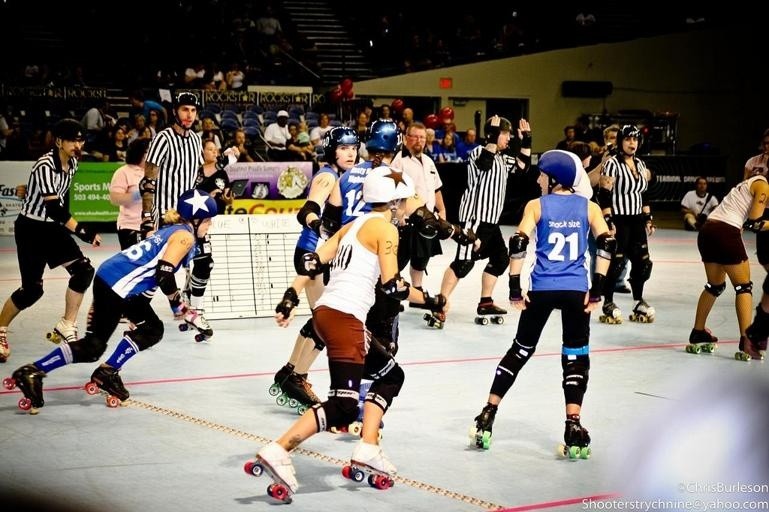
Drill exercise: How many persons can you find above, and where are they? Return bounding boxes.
[1,4,658,364]
[746,129,769,351]
[686,173,769,361]
[2,189,219,416]
[243,167,448,505]
[680,178,720,230]
[471,150,620,463]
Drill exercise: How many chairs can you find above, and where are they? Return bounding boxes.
[199,110,343,136]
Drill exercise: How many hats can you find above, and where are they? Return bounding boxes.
[277,110,289,117]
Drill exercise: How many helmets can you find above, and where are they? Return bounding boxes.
[323,127,360,150]
[363,166,415,203]
[177,189,217,220]
[175,92,200,108]
[537,149,583,186]
[366,118,402,152]
[484,117,512,132]
[53,118,84,141]
[616,123,640,138]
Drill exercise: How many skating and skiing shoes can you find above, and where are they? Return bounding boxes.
[244,442,298,504]
[269,362,383,440]
[735,316,769,361]
[342,442,397,488]
[0,326,9,363]
[475,403,497,449]
[2,364,46,414]
[475,302,507,325]
[686,328,718,353]
[173,302,212,341]
[47,317,77,344]
[558,415,590,460]
[588,285,656,324]
[425,310,446,328]
[85,363,129,407]
